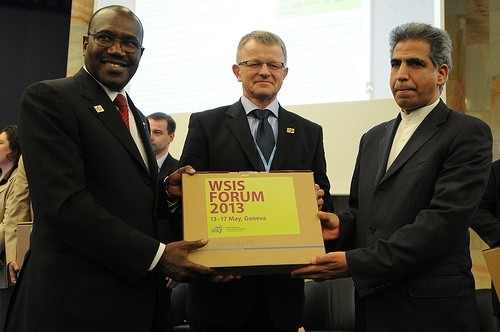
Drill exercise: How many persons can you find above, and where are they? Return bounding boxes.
[0,5,333,332]
[471,160,500,319]
[291,22,493,332]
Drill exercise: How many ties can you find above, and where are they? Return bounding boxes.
[251,109,276,165]
[113,93,130,131]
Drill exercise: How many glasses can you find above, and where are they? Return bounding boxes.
[239,60,285,71]
[87,32,140,54]
